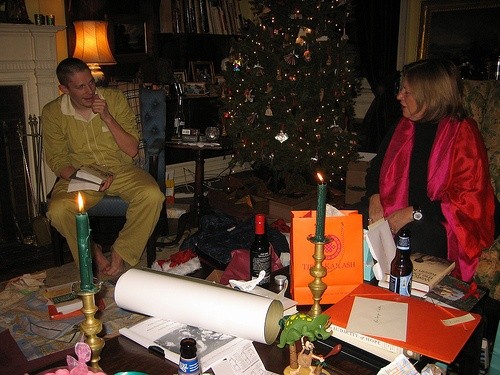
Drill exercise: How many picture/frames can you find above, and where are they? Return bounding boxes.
[172,60,227,98]
[415,0,500,80]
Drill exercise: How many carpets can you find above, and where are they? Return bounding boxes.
[0,226,227,375]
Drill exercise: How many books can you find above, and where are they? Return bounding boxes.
[66,162,113,192]
[43,282,85,319]
[158,0,251,35]
[118,317,254,373]
[320,250,486,364]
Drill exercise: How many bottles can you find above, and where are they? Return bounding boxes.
[171,94,186,140]
[176,338,201,375]
[389,229,413,297]
[251,213,272,289]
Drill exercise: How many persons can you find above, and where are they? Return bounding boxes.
[38,56,166,278]
[203,68,211,81]
[195,67,204,82]
[365,58,500,282]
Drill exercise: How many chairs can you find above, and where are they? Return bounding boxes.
[452,79,500,300]
[47,80,169,267]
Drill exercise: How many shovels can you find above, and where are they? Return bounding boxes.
[33,136,53,246]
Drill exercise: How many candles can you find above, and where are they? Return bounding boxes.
[316,173,328,242]
[72,190,94,290]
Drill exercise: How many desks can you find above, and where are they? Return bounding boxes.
[157,140,232,248]
[25,268,487,375]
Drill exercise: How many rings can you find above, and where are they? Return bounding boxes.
[368,218,374,223]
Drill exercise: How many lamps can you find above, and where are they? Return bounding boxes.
[72,19,115,82]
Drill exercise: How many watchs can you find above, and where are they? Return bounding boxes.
[412,203,423,222]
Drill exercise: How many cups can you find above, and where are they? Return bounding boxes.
[205,127,220,142]
[181,128,199,142]
[33,14,55,25]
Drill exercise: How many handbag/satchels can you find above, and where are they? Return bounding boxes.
[363,228,375,282]
[223,170,264,196]
[191,208,291,273]
[289,210,364,306]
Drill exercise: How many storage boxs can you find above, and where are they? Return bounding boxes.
[165,170,177,205]
[346,151,376,205]
[268,185,332,223]
